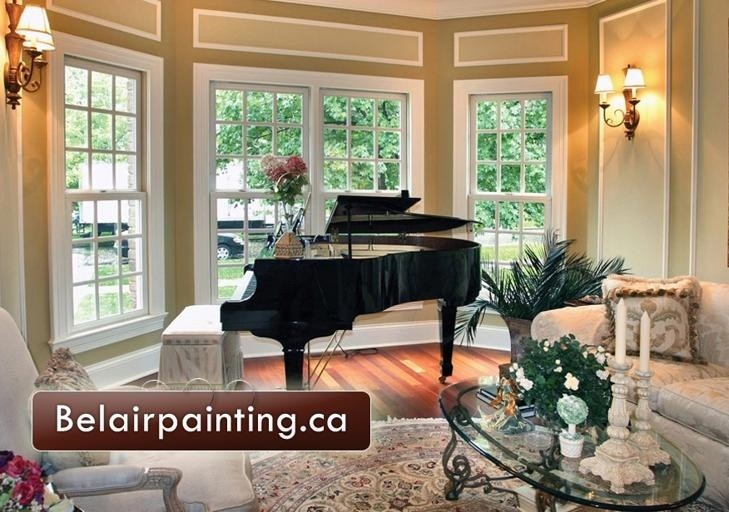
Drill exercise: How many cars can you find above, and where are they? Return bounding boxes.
[78,219,266,265]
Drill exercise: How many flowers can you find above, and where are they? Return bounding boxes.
[261,152,309,231]
[0,448,75,512]
[508,331,611,433]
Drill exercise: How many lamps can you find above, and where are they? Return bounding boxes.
[5,2,56,110]
[594,64,647,141]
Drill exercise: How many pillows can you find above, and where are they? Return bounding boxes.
[34,344,112,470]
[600,272,706,365]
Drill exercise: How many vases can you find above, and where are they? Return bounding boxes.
[273,230,304,260]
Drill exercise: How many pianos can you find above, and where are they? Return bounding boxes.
[220,190,481,390]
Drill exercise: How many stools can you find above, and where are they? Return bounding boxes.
[155,305,243,390]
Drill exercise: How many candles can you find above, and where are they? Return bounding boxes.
[613,296,627,364]
[639,310,651,373]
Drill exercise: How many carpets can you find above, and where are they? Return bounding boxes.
[245,418,717,511]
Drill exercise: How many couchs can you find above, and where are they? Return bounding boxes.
[529,282,729,511]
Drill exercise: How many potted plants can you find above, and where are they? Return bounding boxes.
[452,229,633,362]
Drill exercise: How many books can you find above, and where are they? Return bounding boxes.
[479,382,537,411]
[475,391,536,419]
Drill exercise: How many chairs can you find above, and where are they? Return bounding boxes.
[1,308,262,512]
